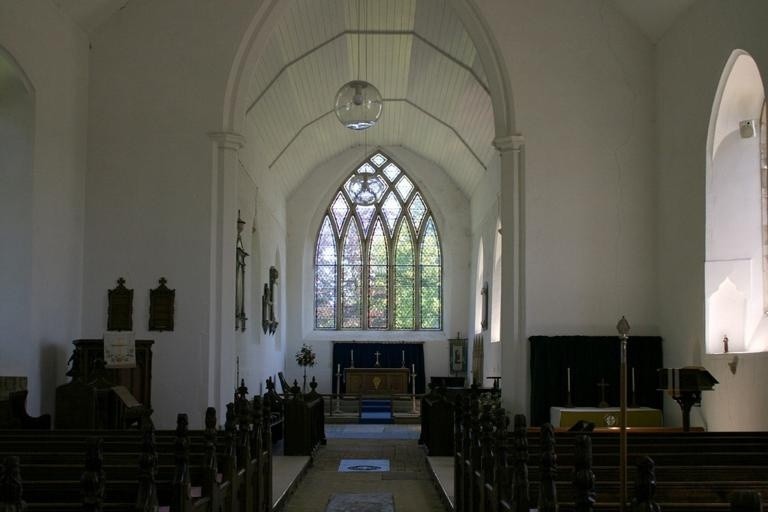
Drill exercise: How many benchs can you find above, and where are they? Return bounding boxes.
[0,392,271,512]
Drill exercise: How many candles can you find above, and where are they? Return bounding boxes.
[336,350,416,395]
[632,367,635,392]
[568,368,570,392]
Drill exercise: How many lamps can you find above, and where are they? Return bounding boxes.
[333,0,383,131]
[348,0,382,205]
